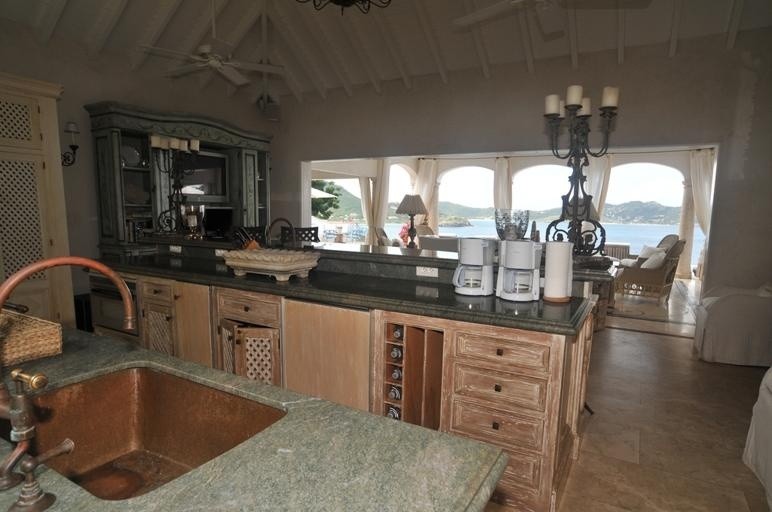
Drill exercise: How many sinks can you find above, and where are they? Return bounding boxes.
[0,359,289,501]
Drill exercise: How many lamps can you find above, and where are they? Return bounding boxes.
[396,195,428,249]
[543,84,619,270]
[150,133,199,238]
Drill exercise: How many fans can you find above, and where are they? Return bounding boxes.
[139,1,284,87]
[455,0,652,38]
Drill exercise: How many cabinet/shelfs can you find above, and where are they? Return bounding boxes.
[372,288,601,512]
[87,260,175,360]
[83,100,271,258]
[174,274,371,411]
[0,72,77,331]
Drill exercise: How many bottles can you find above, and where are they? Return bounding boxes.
[126,219,136,243]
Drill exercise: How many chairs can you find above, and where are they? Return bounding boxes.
[228,225,269,250]
[603,235,686,309]
[281,226,318,248]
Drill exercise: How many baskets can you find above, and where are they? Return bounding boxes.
[0,309,63,369]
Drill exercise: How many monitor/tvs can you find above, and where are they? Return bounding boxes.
[169,150,230,202]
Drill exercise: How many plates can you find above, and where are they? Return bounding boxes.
[123,145,140,167]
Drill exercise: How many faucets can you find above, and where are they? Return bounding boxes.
[0,254,138,334]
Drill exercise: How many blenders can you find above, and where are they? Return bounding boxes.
[452,237,493,296]
[495,240,542,302]
